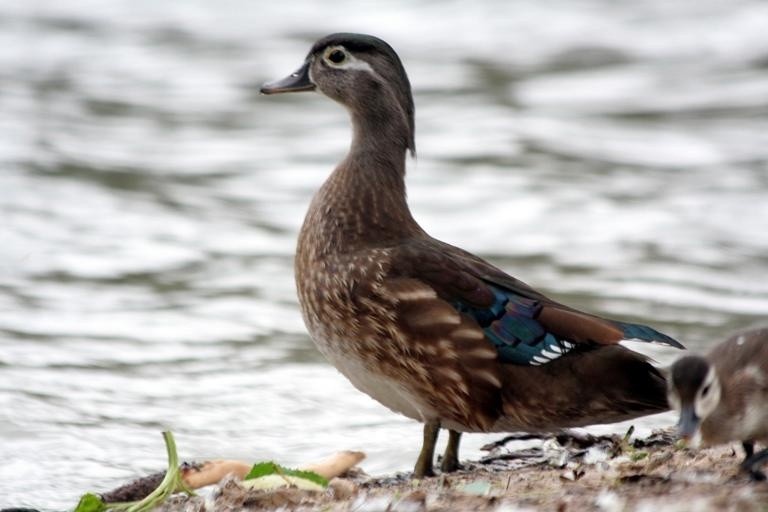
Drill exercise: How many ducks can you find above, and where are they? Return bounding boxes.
[667,328,768,481]
[259,32,686,480]
